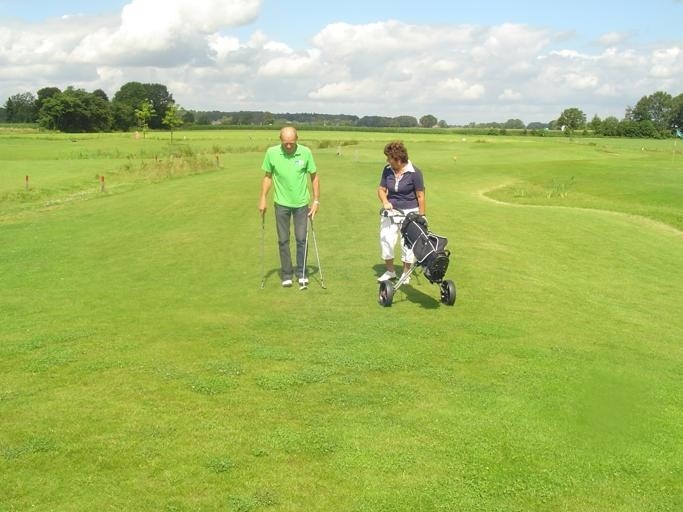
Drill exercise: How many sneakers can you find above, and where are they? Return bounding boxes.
[400,272,410,285]
[281,280,293,287]
[299,276,309,286]
[377,270,396,282]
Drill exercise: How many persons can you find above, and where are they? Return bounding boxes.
[377,141,427,283]
[258,126,321,286]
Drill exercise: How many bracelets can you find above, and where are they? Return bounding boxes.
[313,200,320,204]
[419,214,425,216]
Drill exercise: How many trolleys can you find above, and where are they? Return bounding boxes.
[379,207,456,307]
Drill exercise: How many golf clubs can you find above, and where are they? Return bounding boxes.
[298,206,309,290]
[257,210,267,289]
[308,207,327,289]
